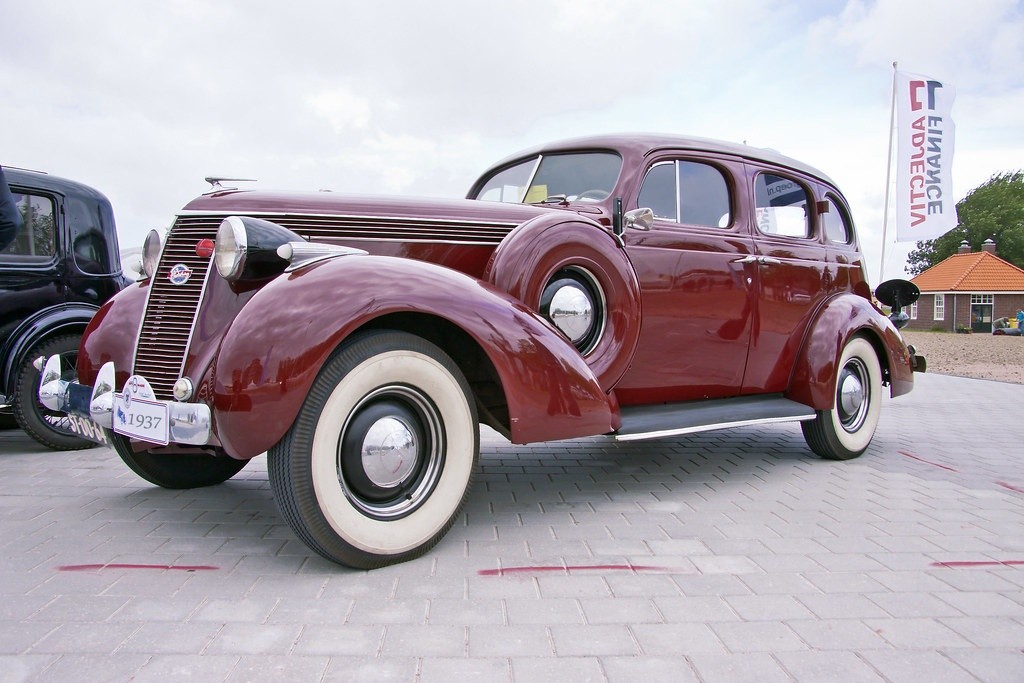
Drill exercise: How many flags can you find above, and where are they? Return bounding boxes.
[894,66,958,242]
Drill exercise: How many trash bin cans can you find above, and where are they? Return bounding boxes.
[1008,318,1019,329]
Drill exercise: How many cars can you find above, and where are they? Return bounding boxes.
[38,127,927,570]
[117,245,146,279]
[0,166,127,451]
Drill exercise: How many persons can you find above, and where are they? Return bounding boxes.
[1016,308,1024,336]
[993,316,1009,329]
